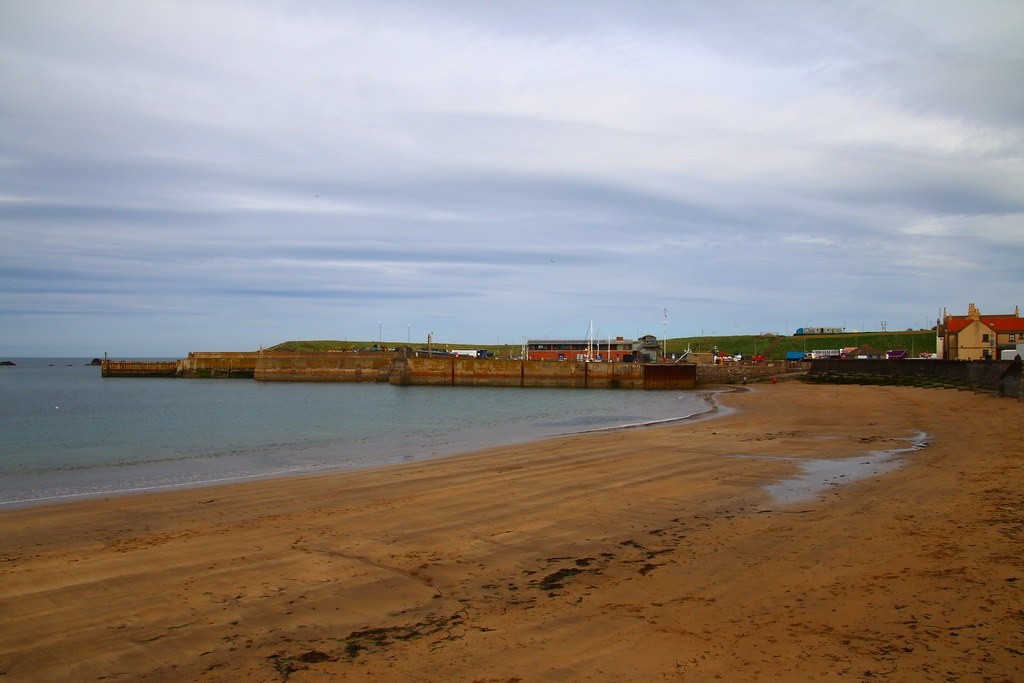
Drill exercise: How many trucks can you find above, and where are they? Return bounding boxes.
[786,352,817,361]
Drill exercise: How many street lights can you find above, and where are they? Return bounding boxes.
[755,339,757,357]
[990,324,994,360]
[378,321,382,342]
[407,324,411,342]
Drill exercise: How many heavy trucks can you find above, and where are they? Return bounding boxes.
[796,328,843,335]
[813,347,858,360]
